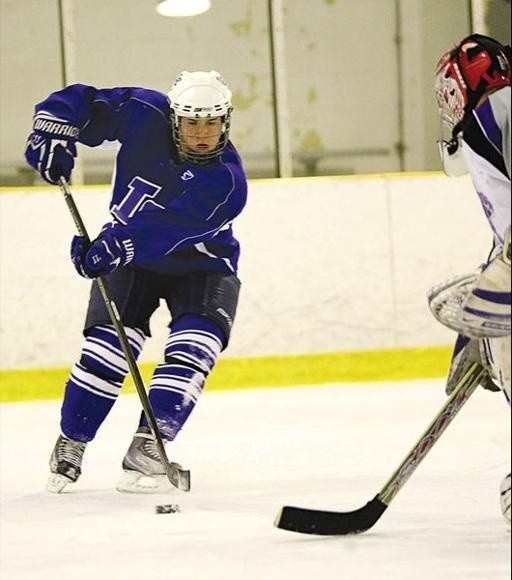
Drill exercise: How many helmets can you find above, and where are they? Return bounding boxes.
[434,33,511,135]
[166,71,235,166]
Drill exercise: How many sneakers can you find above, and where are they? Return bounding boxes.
[48,433,89,484]
[121,425,183,480]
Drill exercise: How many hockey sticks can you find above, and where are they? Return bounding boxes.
[272,365,488,534]
[58,176,191,493]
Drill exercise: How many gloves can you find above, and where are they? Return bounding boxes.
[23,104,81,186]
[70,220,136,281]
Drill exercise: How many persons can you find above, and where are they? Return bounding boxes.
[420,34,511,530]
[20,68,252,481]
